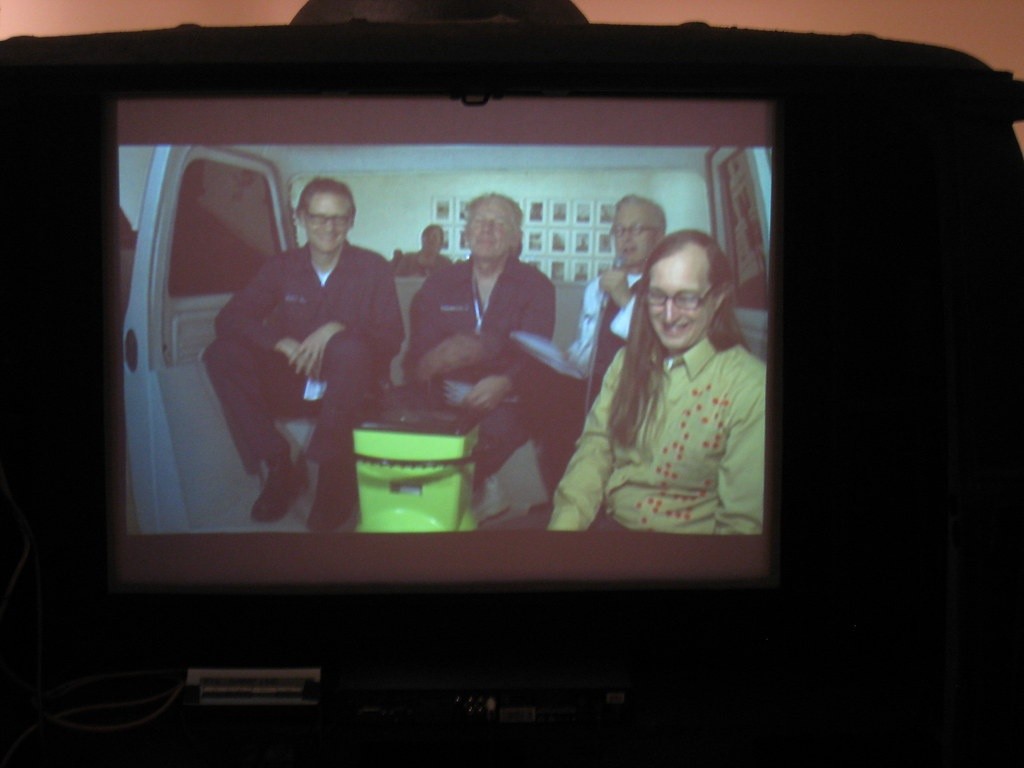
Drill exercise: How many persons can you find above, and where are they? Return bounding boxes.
[374,191,557,520]
[496,227,767,535]
[395,223,452,278]
[528,193,667,525]
[197,177,404,532]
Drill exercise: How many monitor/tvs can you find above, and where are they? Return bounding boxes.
[106,96,785,593]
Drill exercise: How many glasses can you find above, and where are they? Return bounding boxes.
[301,210,351,226]
[645,287,716,308]
[610,220,657,237]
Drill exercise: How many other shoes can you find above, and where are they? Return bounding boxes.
[472,473,510,525]
[252,467,304,520]
[307,476,355,528]
